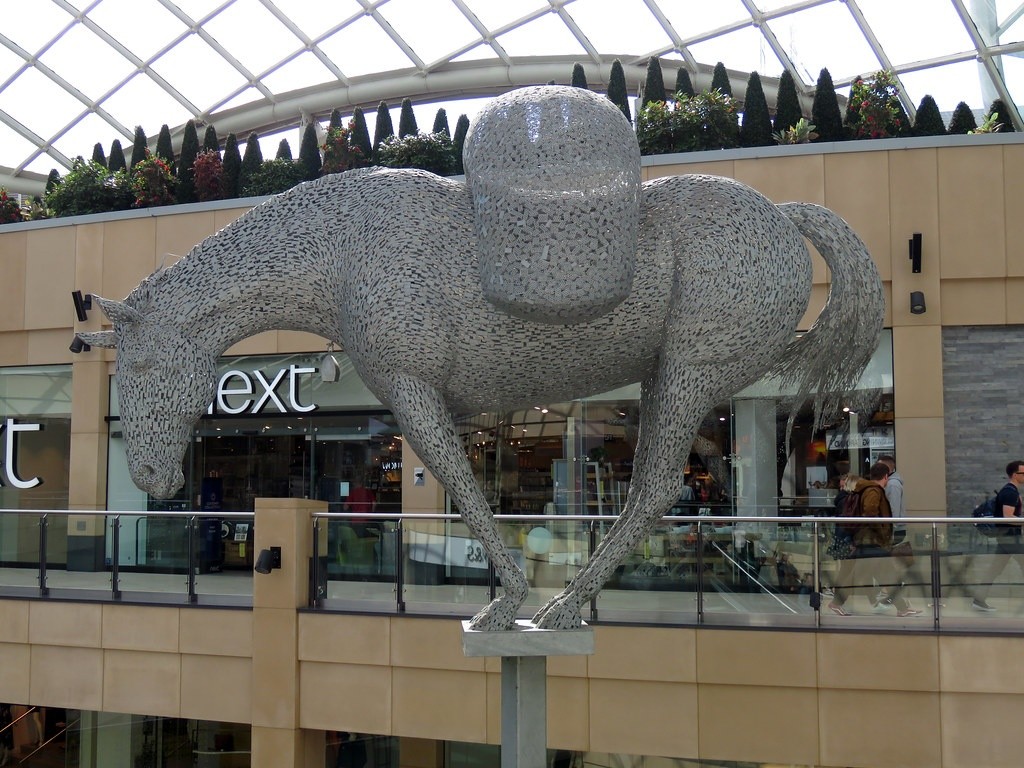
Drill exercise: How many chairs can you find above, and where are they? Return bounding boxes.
[338,526,378,565]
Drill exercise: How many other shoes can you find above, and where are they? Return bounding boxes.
[820,589,835,599]
[828,602,853,616]
[872,603,890,613]
[927,602,945,608]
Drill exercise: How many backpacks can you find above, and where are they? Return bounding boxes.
[972,490,1000,538]
[833,486,868,529]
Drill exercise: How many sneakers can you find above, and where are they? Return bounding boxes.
[896,608,922,616]
[972,599,998,612]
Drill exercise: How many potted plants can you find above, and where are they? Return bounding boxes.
[590,447,608,461]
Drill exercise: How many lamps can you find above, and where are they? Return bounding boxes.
[910,291,926,314]
[70,335,90,353]
[908,232,923,273]
[489,431,495,437]
[72,289,92,322]
[463,436,470,443]
[255,546,281,574]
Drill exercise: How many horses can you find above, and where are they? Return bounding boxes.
[75,167,887,630]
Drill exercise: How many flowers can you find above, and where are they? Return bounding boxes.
[847,69,901,140]
[0,186,23,224]
[129,146,178,209]
[317,117,368,176]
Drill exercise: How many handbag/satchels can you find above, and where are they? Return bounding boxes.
[893,542,915,569]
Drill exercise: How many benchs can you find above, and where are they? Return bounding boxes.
[741,532,841,593]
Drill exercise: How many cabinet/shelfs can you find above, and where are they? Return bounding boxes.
[594,527,733,581]
[587,462,616,542]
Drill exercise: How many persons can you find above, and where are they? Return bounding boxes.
[971,461,1024,612]
[827,457,921,618]
[0,703,14,766]
[30,705,44,748]
[668,484,697,527]
[342,476,378,540]
